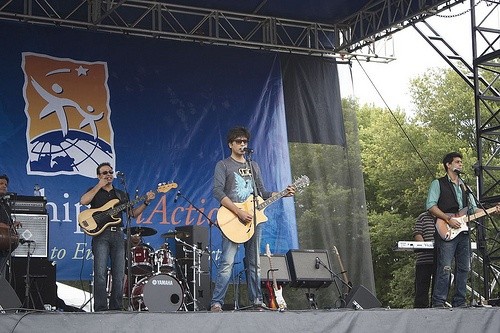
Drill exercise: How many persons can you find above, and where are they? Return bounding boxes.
[210,128,296,314]
[80,162,156,312]
[0,175,23,278]
[412,153,500,308]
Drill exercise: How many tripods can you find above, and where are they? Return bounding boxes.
[230,152,279,313]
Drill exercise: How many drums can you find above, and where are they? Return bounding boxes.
[155,249,175,272]
[129,246,153,277]
[125,273,185,313]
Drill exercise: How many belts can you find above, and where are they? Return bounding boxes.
[105,226,123,232]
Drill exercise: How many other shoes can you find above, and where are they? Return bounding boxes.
[211,302,223,312]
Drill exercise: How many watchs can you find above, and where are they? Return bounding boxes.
[143,200,150,206]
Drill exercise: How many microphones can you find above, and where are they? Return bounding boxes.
[115,171,125,176]
[452,168,465,174]
[174,189,181,203]
[241,147,254,153]
[315,258,320,269]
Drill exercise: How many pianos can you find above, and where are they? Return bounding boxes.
[394,240,479,310]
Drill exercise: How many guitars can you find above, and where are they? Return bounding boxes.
[77,182,179,237]
[330,246,349,299]
[215,174,311,244]
[434,205,500,243]
[265,244,287,310]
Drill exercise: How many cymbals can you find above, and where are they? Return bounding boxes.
[122,227,157,236]
[161,230,194,239]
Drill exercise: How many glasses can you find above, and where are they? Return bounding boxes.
[454,160,462,163]
[100,171,114,175]
[233,139,247,144]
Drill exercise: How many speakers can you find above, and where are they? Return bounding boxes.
[10,214,49,257]
[339,284,382,309]
[176,273,210,311]
[243,254,292,288]
[9,258,56,312]
[286,249,333,288]
[176,225,210,273]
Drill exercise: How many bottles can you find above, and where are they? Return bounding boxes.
[34,183,40,196]
